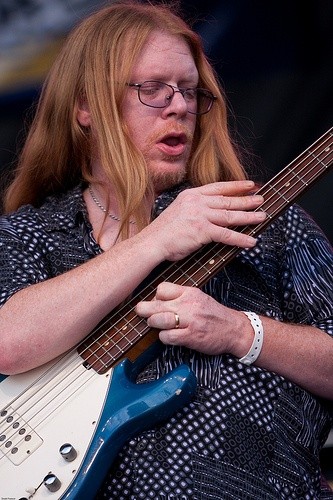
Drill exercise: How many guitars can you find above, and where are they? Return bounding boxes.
[0,127,333,500]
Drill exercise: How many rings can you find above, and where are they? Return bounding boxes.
[174,313,180,329]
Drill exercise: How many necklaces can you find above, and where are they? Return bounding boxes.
[88,185,137,226]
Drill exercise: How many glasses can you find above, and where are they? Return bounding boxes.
[125,74,219,115]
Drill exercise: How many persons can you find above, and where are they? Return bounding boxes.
[0,4,333,500]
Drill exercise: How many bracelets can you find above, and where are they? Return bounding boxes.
[236,311,264,366]
